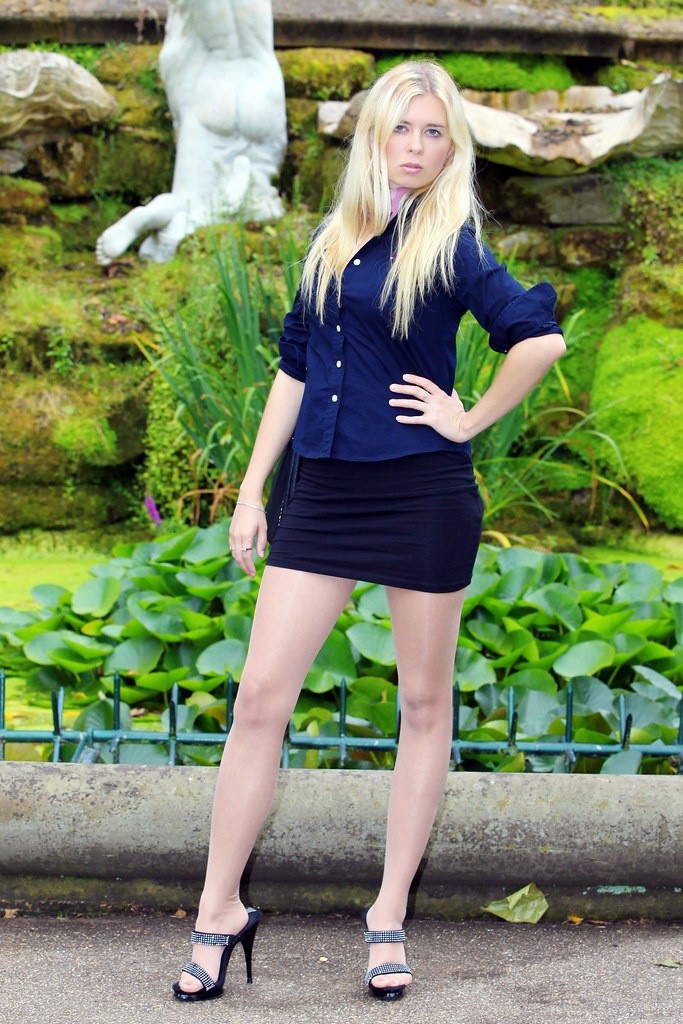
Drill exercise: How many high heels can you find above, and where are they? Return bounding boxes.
[172,905,263,1001]
[363,931,413,1001]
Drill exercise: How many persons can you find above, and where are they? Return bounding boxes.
[170,60,566,999]
[97,1,288,270]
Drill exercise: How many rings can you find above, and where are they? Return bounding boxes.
[229,547,233,550]
[241,547,252,551]
[421,392,428,399]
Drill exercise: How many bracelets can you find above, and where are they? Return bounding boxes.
[235,501,267,514]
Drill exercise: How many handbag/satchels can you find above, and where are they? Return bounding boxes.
[265,434,294,544]
